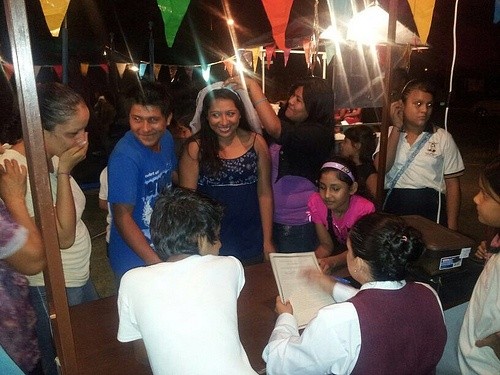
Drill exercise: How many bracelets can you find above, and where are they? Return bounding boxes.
[252,98,268,107]
[55,171,71,178]
[393,124,406,132]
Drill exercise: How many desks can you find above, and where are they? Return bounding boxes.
[69,255,446,375]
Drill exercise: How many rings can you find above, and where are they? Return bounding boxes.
[231,77,234,80]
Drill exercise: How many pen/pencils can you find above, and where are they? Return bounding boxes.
[331,274,351,284]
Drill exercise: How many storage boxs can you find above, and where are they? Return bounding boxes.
[399,215,474,275]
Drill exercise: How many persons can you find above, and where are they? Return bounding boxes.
[0,62,381,375]
[261,210,447,375]
[371,78,465,233]
[456,158,500,375]
